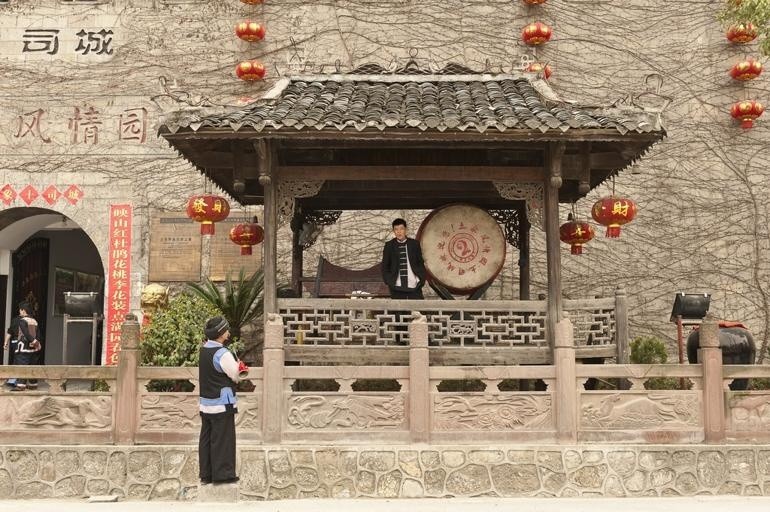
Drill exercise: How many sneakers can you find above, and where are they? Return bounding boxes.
[201,479,212,484]
[4,383,38,392]
[212,477,239,486]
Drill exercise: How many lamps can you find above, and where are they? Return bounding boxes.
[63,292,102,320]
[670,292,711,321]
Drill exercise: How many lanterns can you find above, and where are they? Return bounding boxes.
[520,21,549,47]
[228,223,264,256]
[728,21,760,43]
[185,192,231,235]
[592,197,635,237]
[524,0,548,4]
[730,99,763,129]
[235,22,266,42]
[559,220,595,254]
[235,61,266,80]
[729,60,761,82]
[525,59,551,81]
[241,0,265,6]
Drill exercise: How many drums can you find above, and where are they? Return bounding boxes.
[415,202,506,295]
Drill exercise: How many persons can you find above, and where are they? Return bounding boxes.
[12,304,42,391]
[4,301,29,387]
[196,317,240,485]
[381,218,428,346]
